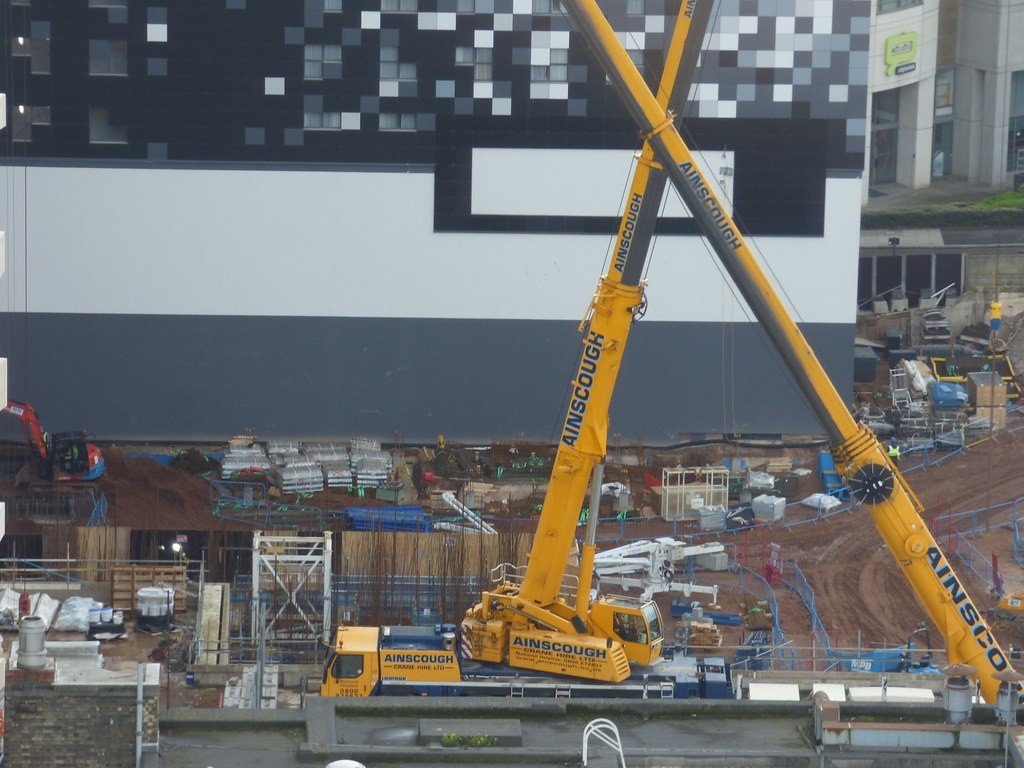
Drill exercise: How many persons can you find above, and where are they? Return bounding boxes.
[886,436,900,467]
[63,441,78,460]
[40,426,50,447]
[614,612,629,634]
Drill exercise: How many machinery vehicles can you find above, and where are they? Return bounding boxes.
[322,0,1023,728]
[3,400,106,483]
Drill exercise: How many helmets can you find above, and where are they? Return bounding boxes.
[890,437,896,444]
